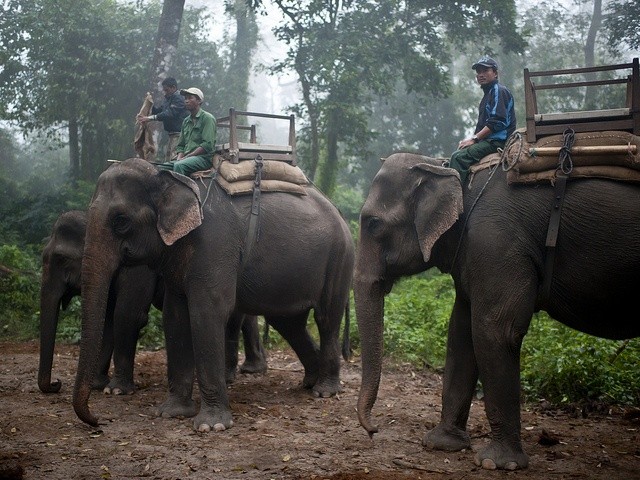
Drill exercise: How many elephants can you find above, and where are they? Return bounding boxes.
[351,152,639,470]
[37,208,269,393]
[72,156,355,433]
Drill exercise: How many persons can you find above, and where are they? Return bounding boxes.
[448,56,516,189]
[136,76,190,162]
[155,86,217,175]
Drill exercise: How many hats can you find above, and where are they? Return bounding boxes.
[471,57,498,70]
[180,87,204,103]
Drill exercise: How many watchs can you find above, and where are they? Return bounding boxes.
[472,134,479,143]
